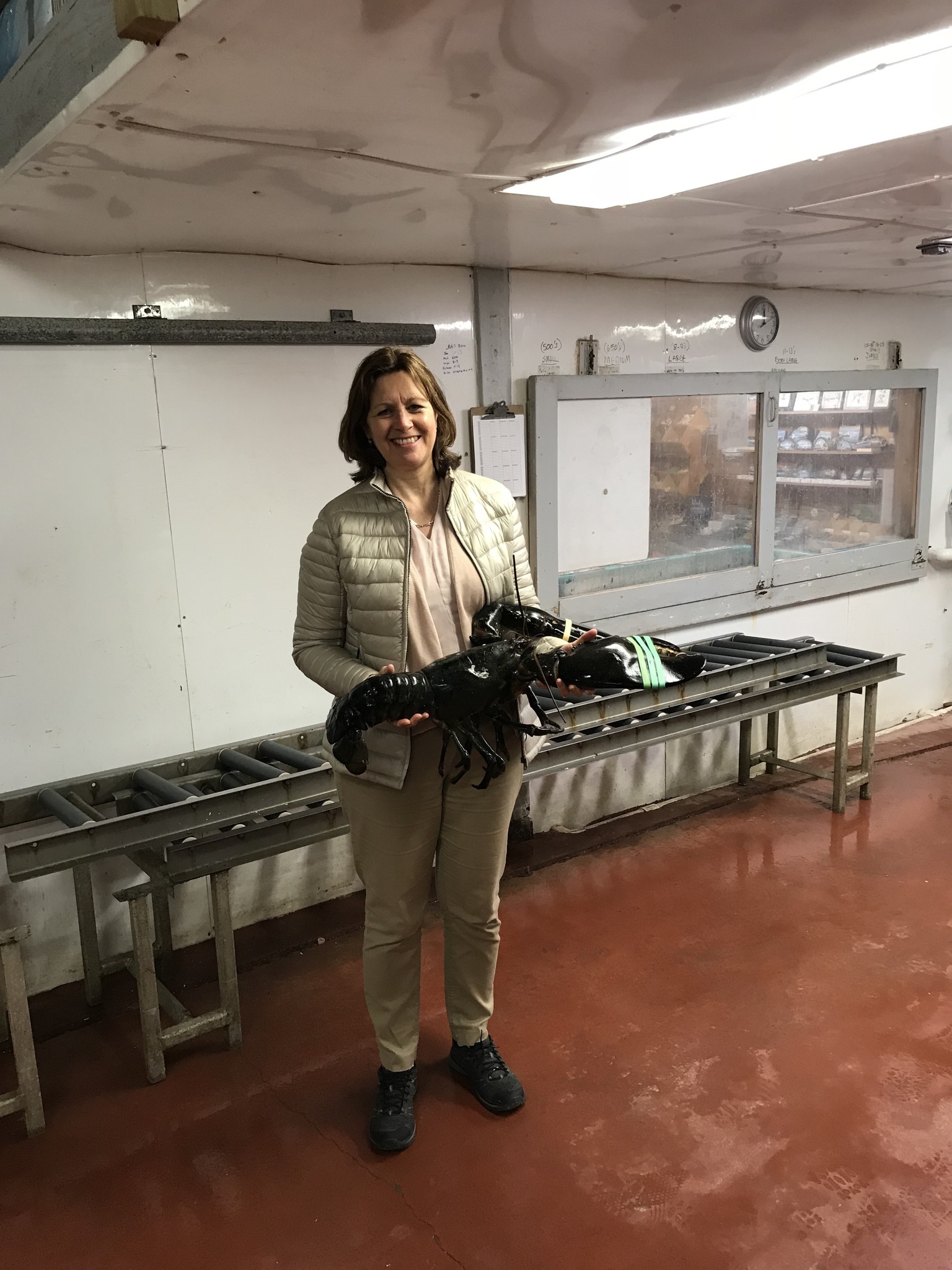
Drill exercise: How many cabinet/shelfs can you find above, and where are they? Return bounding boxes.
[736,410,888,503]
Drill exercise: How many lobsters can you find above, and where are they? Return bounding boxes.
[326,601,706,790]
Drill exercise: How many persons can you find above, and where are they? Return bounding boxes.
[292,346,596,1152]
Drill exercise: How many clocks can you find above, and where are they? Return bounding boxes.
[740,296,779,352]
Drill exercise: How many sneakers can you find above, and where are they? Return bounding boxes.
[369,1061,419,1152]
[447,1029,526,1113]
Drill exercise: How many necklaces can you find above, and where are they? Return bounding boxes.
[409,480,440,528]
